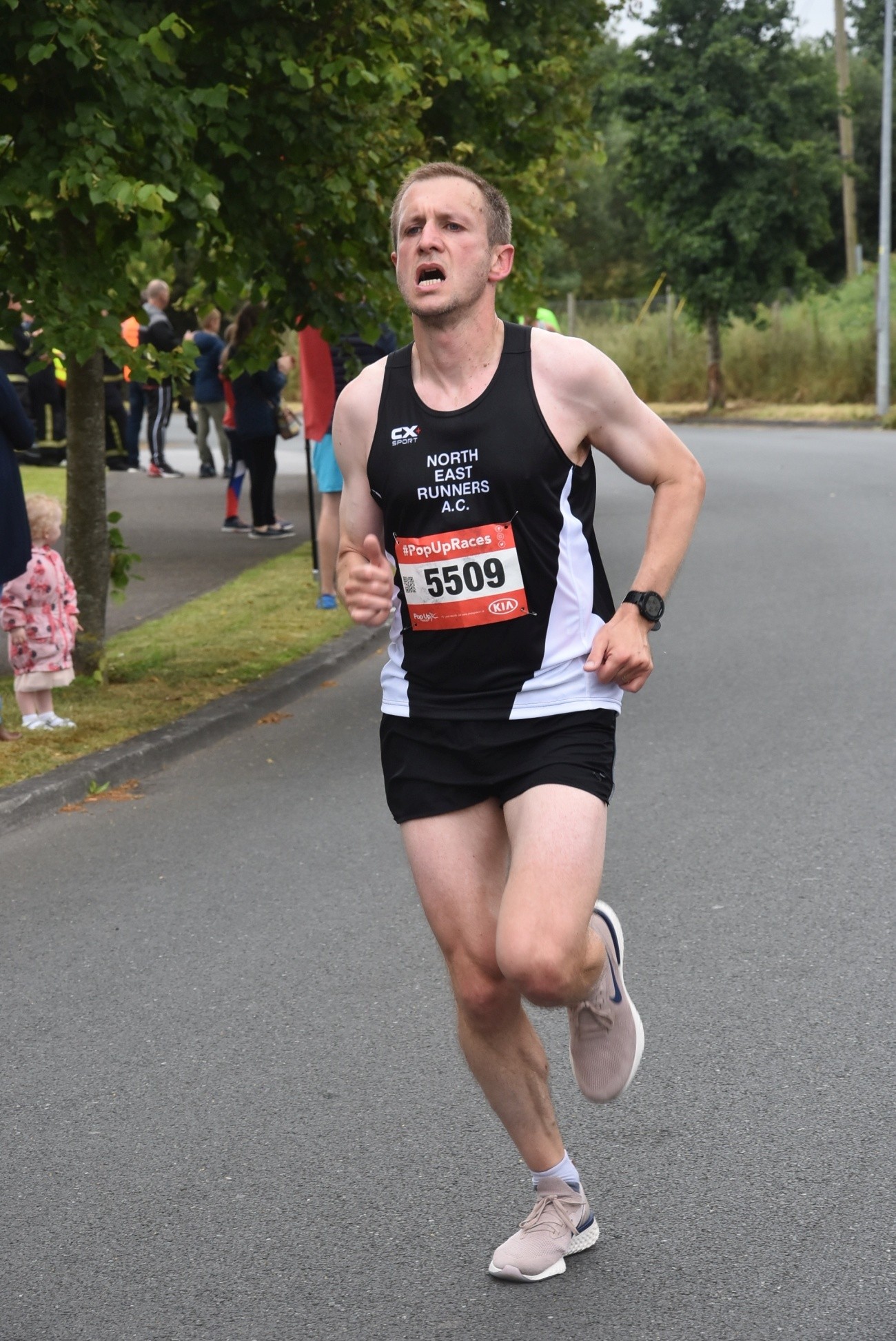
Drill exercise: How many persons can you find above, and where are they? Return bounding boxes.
[294,270,398,609]
[329,161,708,1283]
[0,365,84,742]
[518,307,562,335]
[0,278,193,479]
[189,301,293,540]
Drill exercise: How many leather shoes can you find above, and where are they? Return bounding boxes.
[0,724,22,741]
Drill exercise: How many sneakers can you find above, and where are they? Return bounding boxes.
[488,1175,599,1282]
[566,899,644,1104]
[147,463,185,478]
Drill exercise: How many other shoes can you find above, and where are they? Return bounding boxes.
[315,592,338,610]
[222,465,233,478]
[200,461,217,477]
[248,521,296,539]
[221,516,253,532]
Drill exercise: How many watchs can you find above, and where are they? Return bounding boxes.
[622,591,665,631]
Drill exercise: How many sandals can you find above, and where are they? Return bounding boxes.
[21,717,54,730]
[45,714,77,727]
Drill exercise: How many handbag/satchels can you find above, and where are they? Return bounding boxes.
[278,409,301,440]
